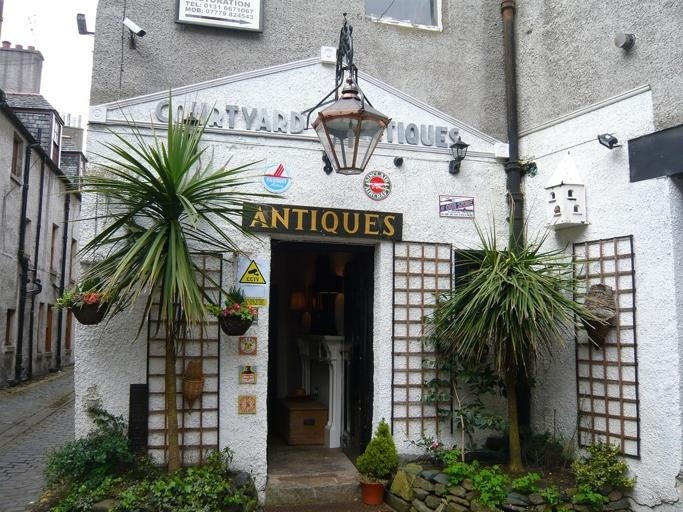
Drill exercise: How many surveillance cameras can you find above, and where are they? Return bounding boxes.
[123,17,146,42]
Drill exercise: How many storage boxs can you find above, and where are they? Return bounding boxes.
[276,399,329,446]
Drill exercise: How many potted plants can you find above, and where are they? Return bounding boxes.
[351,416,398,507]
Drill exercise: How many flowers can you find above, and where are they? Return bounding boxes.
[212,288,256,318]
[53,270,118,305]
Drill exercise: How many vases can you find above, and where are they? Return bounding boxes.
[66,299,111,328]
[216,318,253,336]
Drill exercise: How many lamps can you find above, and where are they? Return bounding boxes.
[310,12,394,175]
[450,136,470,164]
[596,131,619,154]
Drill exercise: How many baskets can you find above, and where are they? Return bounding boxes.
[181,376,204,413]
